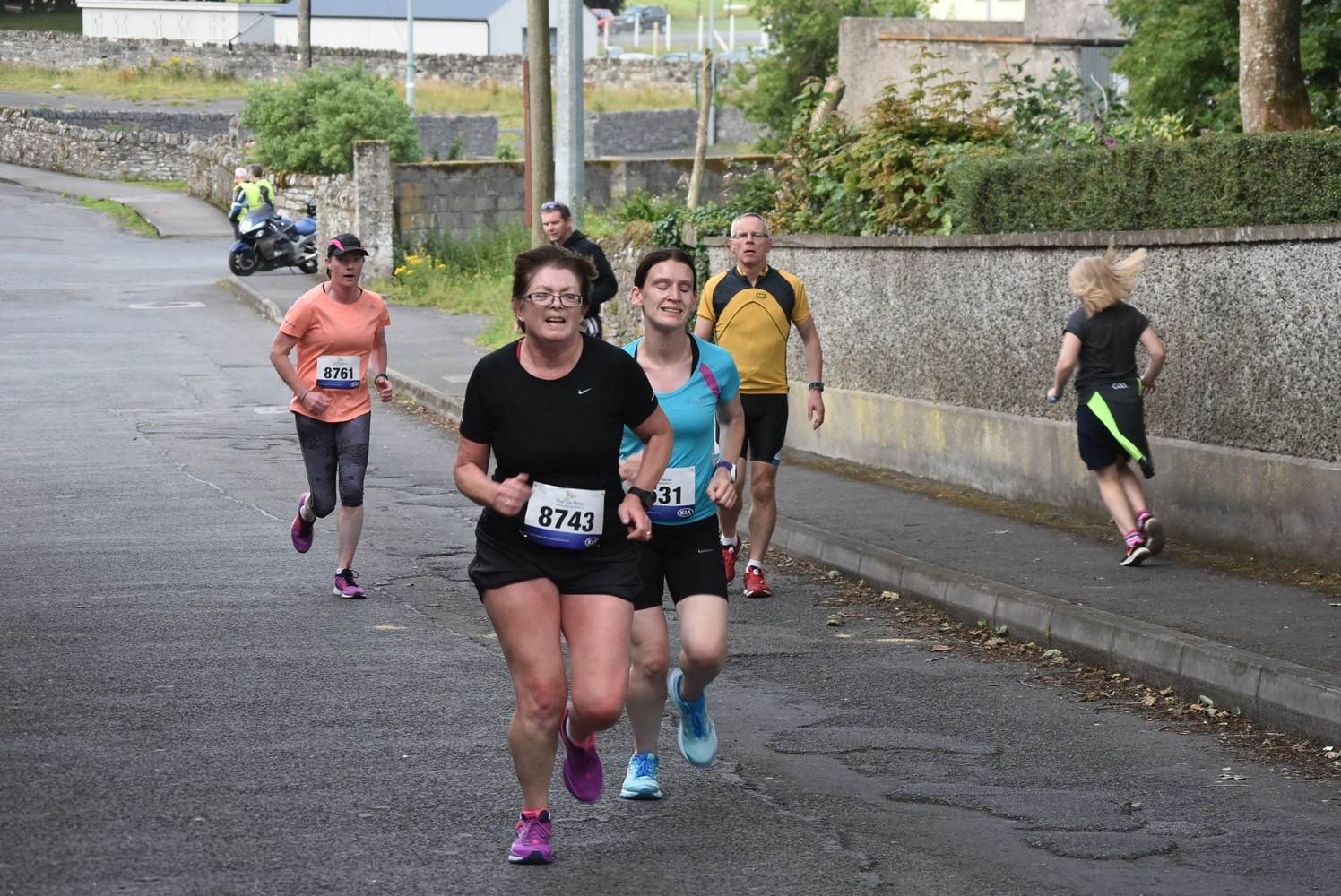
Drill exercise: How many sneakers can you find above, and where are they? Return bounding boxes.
[621,749,663,801]
[559,702,604,804]
[508,806,553,863]
[720,532,742,585]
[669,667,719,766]
[333,562,367,599]
[1139,515,1165,555]
[1120,539,1150,566]
[741,564,774,597]
[290,492,316,553]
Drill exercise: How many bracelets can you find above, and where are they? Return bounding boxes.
[298,390,310,403]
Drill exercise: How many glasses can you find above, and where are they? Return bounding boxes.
[541,202,562,211]
[517,292,584,307]
[733,231,768,241]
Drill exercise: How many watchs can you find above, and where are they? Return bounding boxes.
[713,461,738,483]
[809,382,824,393]
[374,372,388,386]
[627,486,658,510]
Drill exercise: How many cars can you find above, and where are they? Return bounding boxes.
[590,8,616,36]
[611,5,668,34]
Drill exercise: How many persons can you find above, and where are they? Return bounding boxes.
[542,202,617,343]
[228,165,274,243]
[619,248,744,801]
[268,232,393,599]
[1046,235,1166,566]
[453,240,675,863]
[691,212,825,599]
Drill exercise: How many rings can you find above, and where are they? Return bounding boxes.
[646,531,651,535]
[504,499,510,506]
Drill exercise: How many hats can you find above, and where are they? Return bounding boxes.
[328,234,369,260]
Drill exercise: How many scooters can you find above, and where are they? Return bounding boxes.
[229,186,318,276]
